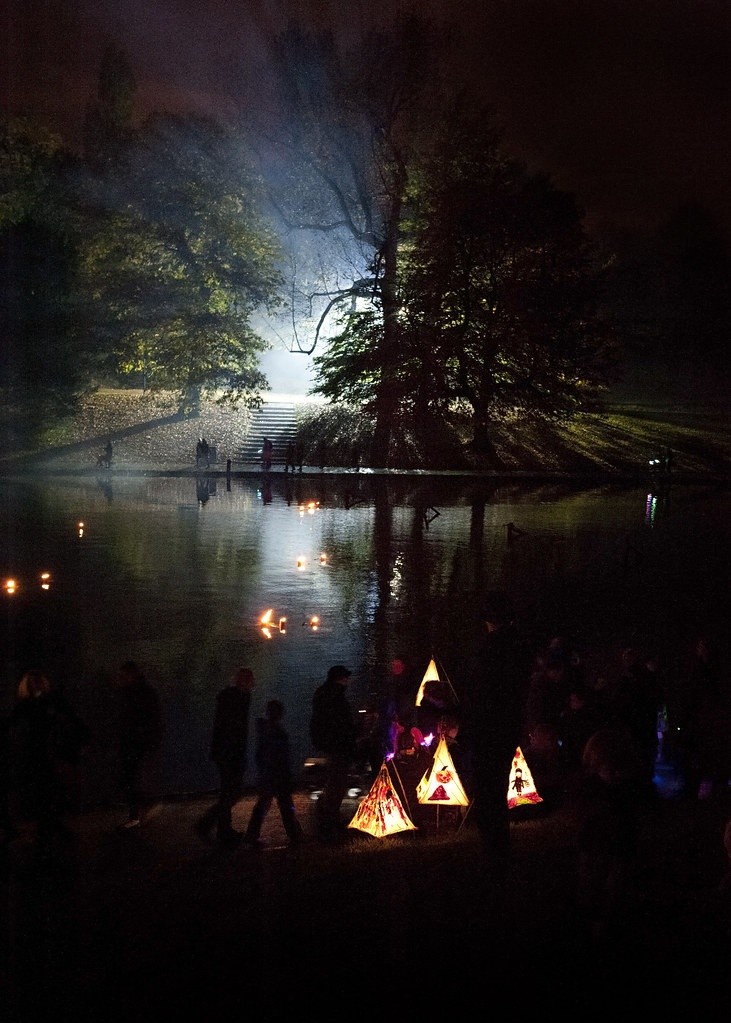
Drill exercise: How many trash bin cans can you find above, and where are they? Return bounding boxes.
[209,446,217,462]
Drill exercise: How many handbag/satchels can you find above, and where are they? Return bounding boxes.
[344,740,369,775]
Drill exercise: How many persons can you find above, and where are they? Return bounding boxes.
[99,438,374,473]
[12,589,731,951]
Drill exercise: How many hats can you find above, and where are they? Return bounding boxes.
[327,665,352,681]
